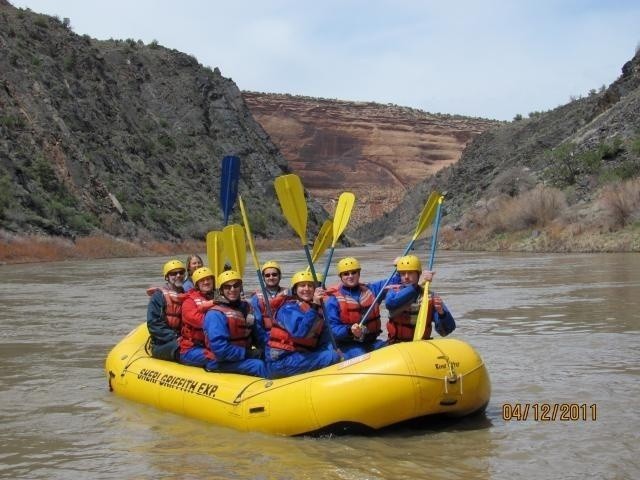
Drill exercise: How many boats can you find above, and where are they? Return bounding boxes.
[104,317,491,437]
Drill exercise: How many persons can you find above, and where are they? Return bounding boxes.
[203,270,266,377]
[251,261,292,351]
[178,267,221,371]
[322,256,401,359]
[182,255,204,293]
[148,260,187,363]
[265,271,340,379]
[379,256,456,347]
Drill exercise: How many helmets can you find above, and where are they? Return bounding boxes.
[163,255,422,292]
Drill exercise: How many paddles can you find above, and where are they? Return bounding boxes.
[237,192,272,317]
[414,196,447,340]
[224,223,247,281]
[207,230,225,278]
[359,190,439,327]
[220,156,241,225]
[274,174,355,361]
